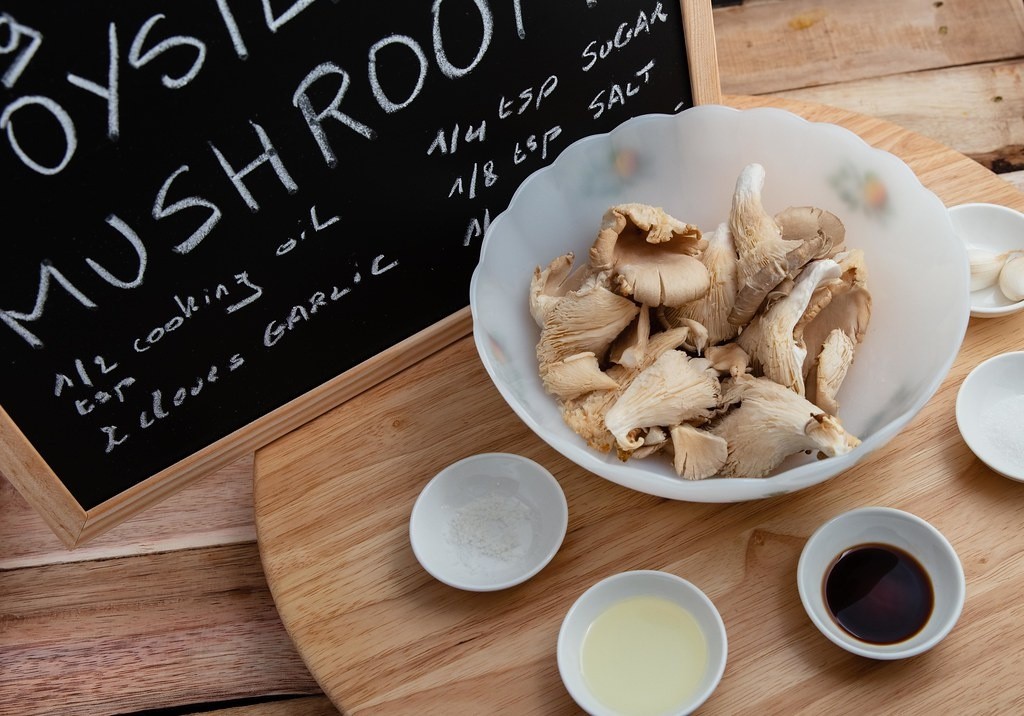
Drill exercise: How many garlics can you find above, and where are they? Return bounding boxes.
[969,249,1024,302]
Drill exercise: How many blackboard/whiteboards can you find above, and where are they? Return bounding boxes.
[0,1,724,553]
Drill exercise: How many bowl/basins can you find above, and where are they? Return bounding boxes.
[470,103,971,502]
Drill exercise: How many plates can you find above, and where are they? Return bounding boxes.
[796,508,968,660]
[556,570,727,715]
[947,203,1024,319]
[955,351,1023,484]
[409,454,568,592]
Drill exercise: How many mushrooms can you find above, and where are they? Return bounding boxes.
[528,164,872,481]
